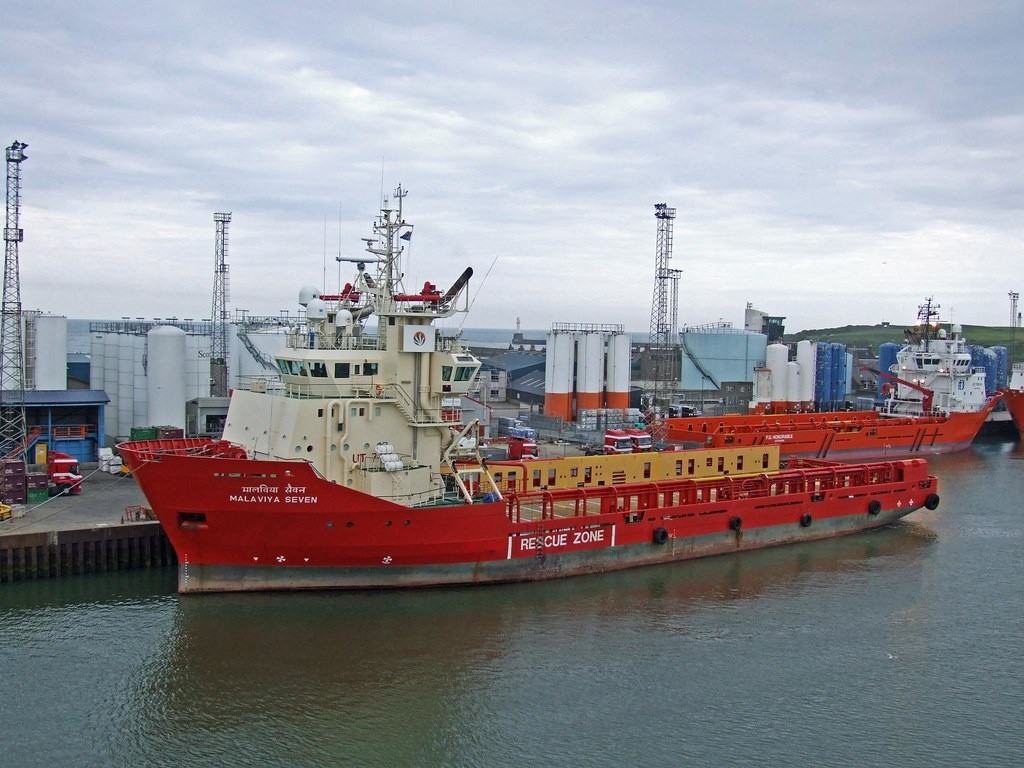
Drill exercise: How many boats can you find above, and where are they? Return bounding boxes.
[115,157,939,594]
[647,297,1004,461]
[995,363,1024,432]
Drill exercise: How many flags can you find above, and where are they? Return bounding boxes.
[400,231,412,241]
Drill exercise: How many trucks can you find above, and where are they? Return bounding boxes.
[625,428,653,453]
[584,429,633,455]
[49,450,84,496]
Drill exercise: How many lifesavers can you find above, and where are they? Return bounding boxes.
[375,385,381,396]
[882,383,893,395]
